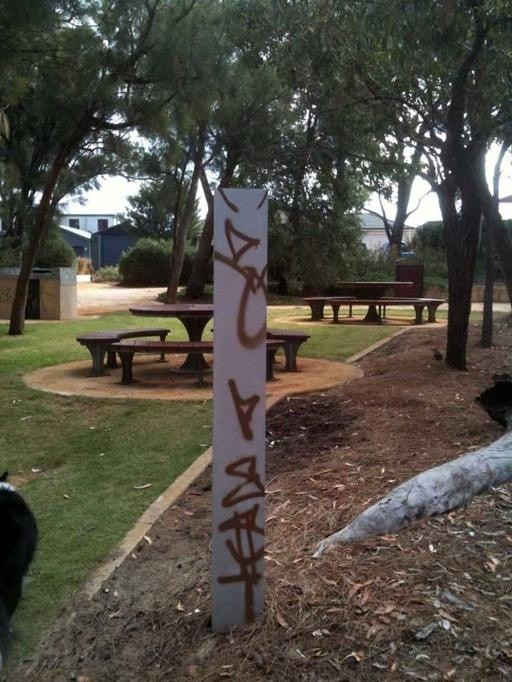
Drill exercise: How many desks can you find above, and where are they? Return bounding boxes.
[334,281,414,321]
[129,305,214,368]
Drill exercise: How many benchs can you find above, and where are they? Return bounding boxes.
[302,296,356,320]
[328,300,431,325]
[76,329,170,377]
[111,341,285,382]
[381,296,445,322]
[211,329,310,371]
